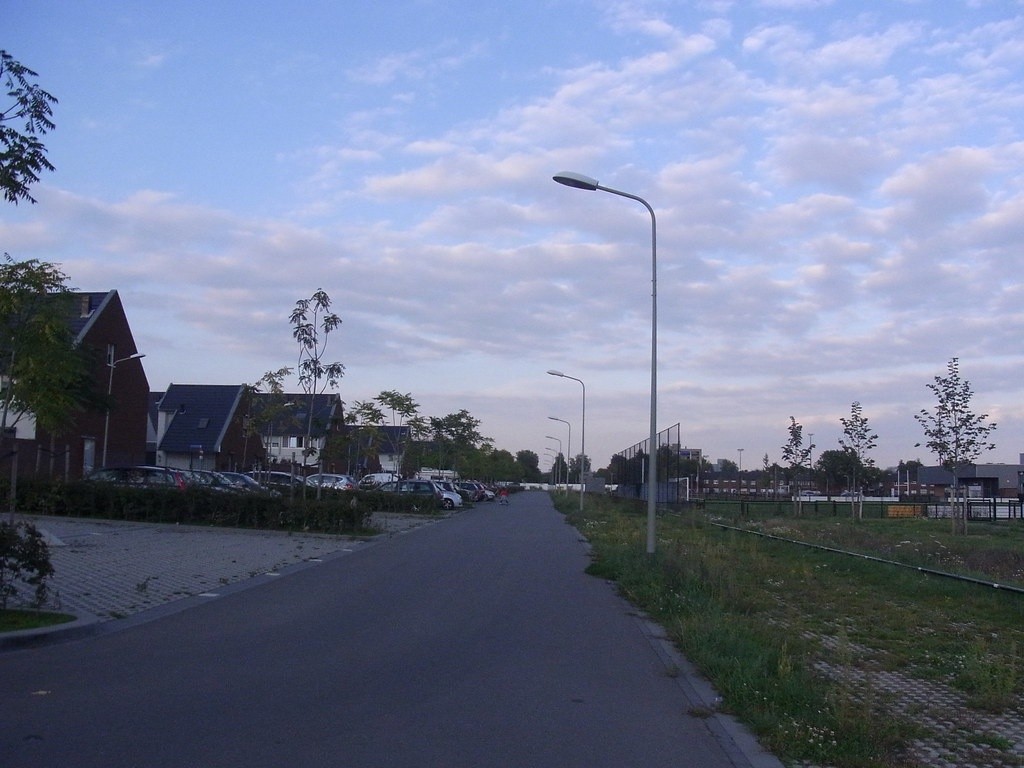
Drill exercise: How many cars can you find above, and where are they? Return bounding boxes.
[79,465,495,511]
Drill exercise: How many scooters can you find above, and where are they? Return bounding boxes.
[499,495,509,506]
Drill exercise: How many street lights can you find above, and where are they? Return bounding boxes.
[543,435,561,495]
[547,370,586,511]
[547,417,571,499]
[102,353,146,469]
[551,170,657,554]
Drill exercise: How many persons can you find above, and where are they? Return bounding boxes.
[499,487,510,505]
